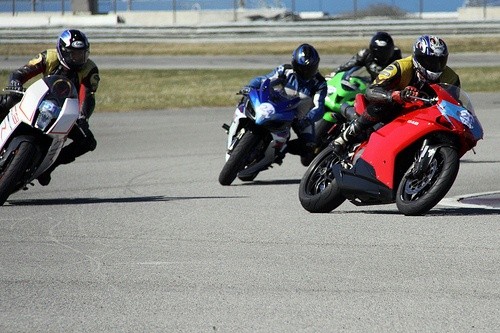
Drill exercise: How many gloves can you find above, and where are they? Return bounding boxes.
[76,118,89,133]
[7,79,23,96]
[330,67,342,77]
[392,86,419,105]
[292,116,312,132]
[240,85,254,97]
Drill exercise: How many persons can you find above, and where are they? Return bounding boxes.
[329,34,461,160]
[335,31,401,84]
[0,30,100,186]
[240,43,328,154]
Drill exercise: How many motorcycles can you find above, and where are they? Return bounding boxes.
[300,64,376,167]
[217,77,302,186]
[0,74,80,206]
[298,82,484,216]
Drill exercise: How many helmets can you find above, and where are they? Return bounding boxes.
[370,31,394,62]
[412,34,449,82]
[56,29,90,70]
[291,43,320,80]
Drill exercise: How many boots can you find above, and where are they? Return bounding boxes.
[330,118,368,151]
[38,145,75,185]
